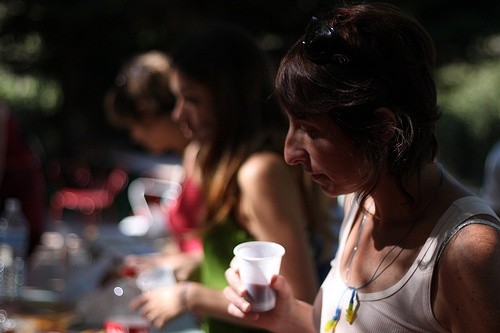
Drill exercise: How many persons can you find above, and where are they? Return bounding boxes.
[218,4,498,333]
[122,19,320,332]
[105,46,199,232]
[0,100,47,257]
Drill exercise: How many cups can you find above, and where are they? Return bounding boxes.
[137,266,177,293]
[233,241,288,312]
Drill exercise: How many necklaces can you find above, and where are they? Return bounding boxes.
[322,200,430,333]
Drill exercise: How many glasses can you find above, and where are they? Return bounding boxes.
[302,16,375,80]
